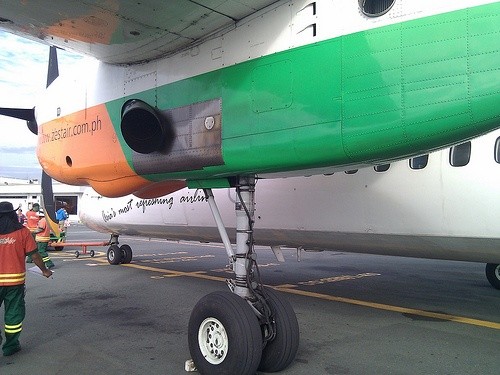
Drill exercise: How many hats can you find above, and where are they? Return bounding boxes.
[0,201,21,212]
[36,209,45,217]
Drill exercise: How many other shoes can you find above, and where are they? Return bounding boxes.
[47,265,55,268]
[3,340,21,356]
[26,261,34,263]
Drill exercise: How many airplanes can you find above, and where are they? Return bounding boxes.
[1,0,499,375]
[78,126,500,291]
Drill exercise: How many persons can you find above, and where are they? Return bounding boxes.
[17,203,55,269]
[55,202,70,237]
[0,202,52,356]
